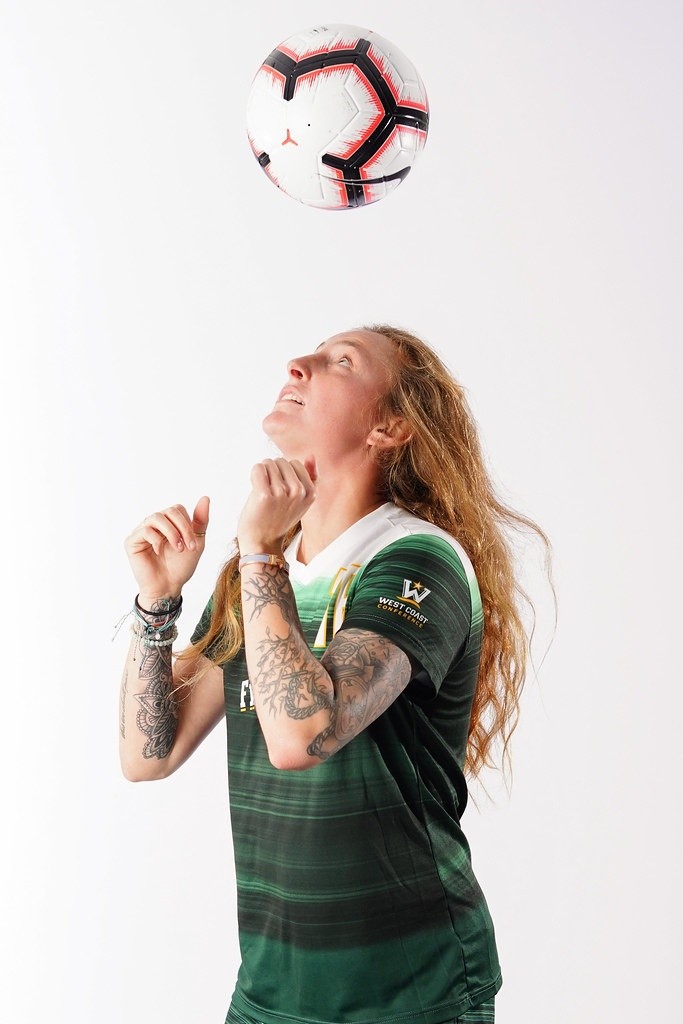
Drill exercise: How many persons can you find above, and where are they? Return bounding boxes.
[108,326,556,1024]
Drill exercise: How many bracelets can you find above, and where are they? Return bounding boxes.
[239,554,289,572]
[112,593,184,660]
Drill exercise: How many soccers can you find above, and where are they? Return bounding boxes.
[242,20,435,213]
